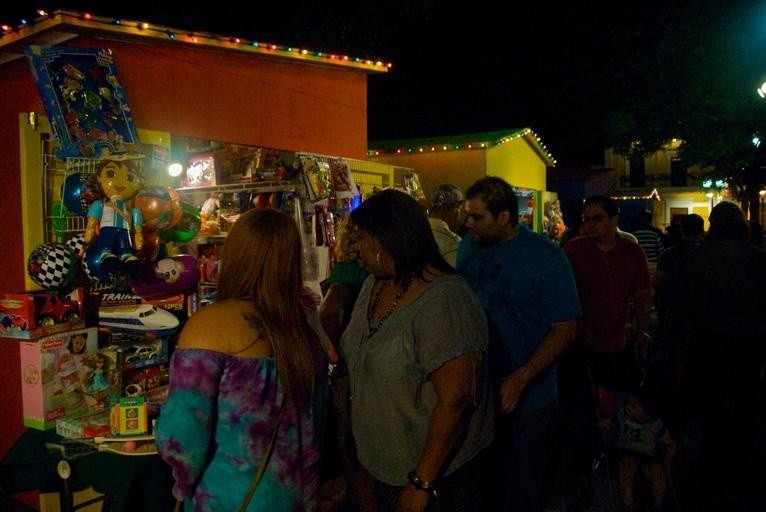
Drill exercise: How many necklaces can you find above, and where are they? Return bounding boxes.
[367,275,415,331]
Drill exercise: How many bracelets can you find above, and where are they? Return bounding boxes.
[408,470,440,498]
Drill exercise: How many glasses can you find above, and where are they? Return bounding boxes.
[581,212,613,223]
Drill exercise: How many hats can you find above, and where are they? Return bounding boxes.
[427,183,467,211]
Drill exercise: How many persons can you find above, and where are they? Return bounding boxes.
[153,208,331,512]
[338,189,499,512]
[318,255,370,352]
[456,176,583,511]
[426,183,463,268]
